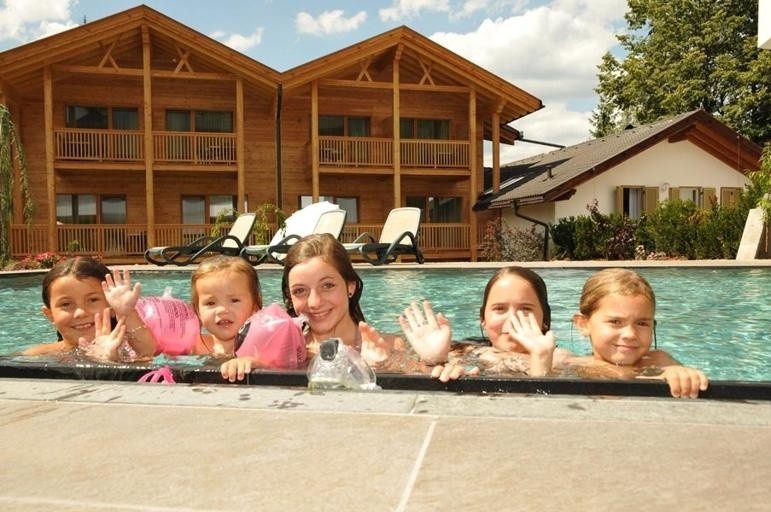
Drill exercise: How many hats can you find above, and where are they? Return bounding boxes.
[307,337,381,393]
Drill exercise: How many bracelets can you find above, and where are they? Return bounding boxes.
[424,361,448,367]
[125,325,147,334]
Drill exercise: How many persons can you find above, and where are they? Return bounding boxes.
[101,255,273,383]
[507,269,709,399]
[282,234,406,374]
[19,257,126,364]
[399,266,574,383]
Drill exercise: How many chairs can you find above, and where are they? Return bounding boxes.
[142,201,425,268]
